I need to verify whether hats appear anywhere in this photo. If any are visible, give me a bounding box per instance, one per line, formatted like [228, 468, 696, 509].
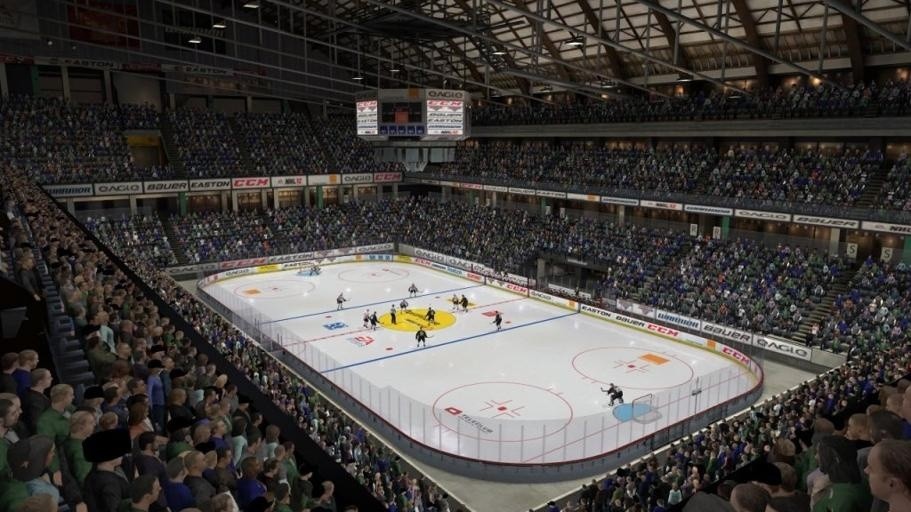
[6, 439, 43, 482]
[148, 360, 165, 368]
[169, 367, 188, 378]
[84, 324, 101, 336]
[151, 345, 166, 353]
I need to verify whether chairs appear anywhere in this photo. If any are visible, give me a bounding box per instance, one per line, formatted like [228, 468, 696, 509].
[1, 214, 96, 404]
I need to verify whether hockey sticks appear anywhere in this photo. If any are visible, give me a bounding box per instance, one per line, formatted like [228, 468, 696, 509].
[601, 387, 609, 392]
